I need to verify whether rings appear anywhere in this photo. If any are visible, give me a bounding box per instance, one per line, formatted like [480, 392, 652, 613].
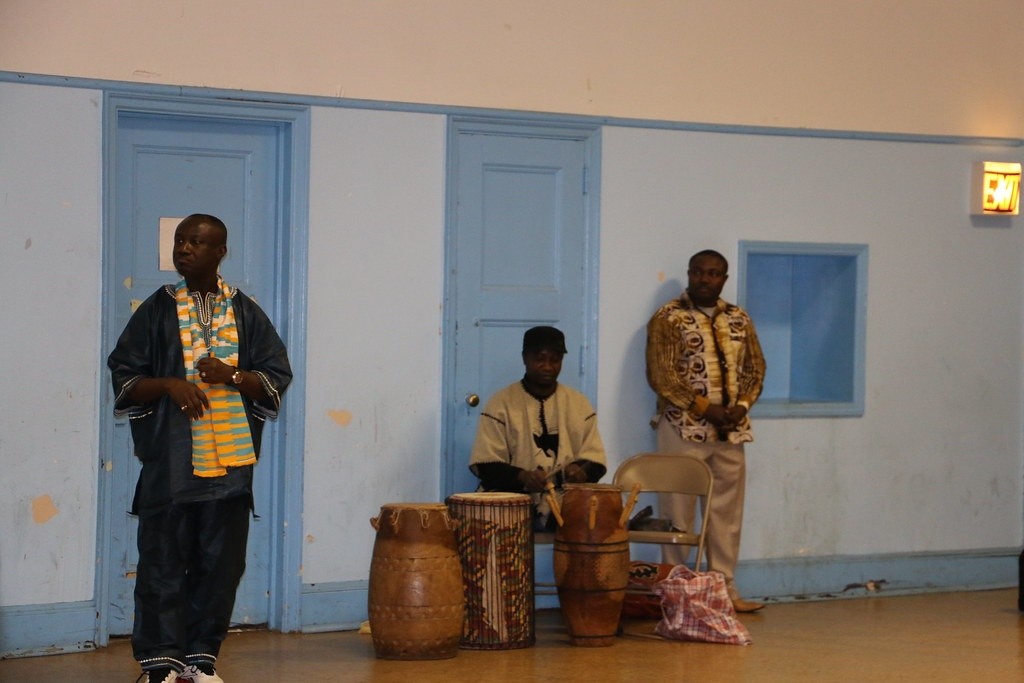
[201, 372, 206, 377]
[181, 404, 187, 410]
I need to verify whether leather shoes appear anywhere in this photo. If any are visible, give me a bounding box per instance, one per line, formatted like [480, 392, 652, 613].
[731, 598, 764, 613]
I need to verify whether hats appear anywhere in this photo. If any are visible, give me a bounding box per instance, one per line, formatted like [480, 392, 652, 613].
[522, 326, 570, 354]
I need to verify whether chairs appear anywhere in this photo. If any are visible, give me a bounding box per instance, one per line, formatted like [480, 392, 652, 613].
[610, 453, 713, 642]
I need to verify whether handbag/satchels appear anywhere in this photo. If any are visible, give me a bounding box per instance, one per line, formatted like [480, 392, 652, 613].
[653, 563, 753, 645]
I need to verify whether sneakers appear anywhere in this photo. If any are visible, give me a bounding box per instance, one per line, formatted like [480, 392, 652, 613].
[145, 664, 224, 683]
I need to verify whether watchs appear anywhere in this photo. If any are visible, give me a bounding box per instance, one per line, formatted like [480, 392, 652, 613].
[225, 365, 244, 387]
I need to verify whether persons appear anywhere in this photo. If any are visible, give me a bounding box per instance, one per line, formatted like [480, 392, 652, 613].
[469, 325, 608, 535]
[644, 249, 766, 612]
[106, 211, 294, 683]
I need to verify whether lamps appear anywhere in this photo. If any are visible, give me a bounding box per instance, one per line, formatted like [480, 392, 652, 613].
[969, 160, 1024, 217]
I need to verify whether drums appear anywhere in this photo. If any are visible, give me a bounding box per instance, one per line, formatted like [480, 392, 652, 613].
[364, 503, 465, 660]
[445, 491, 537, 650]
[541, 482, 642, 647]
